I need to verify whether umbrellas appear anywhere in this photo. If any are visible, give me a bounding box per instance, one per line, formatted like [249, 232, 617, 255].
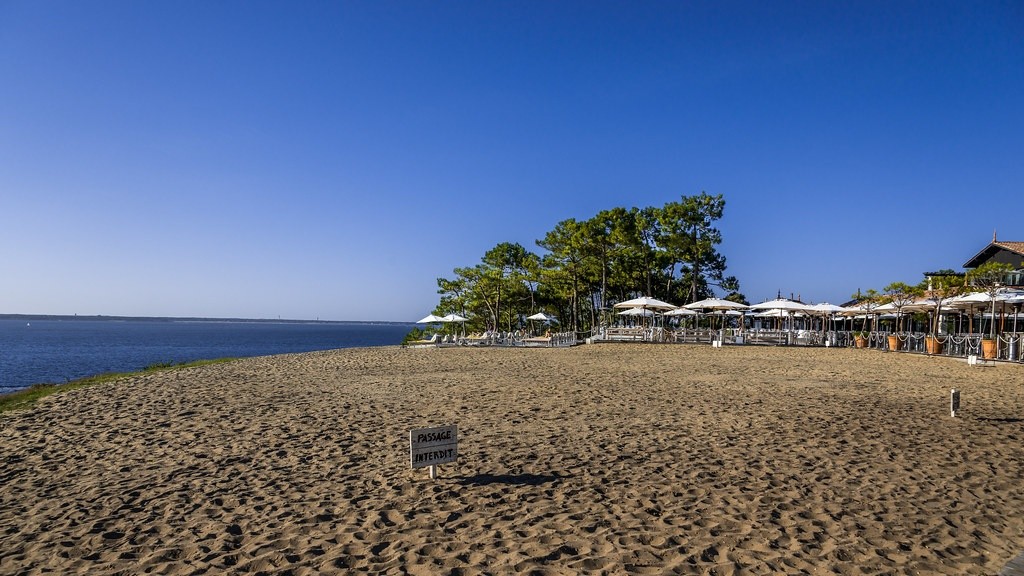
[614, 288, 1024, 338]
[526, 312, 553, 336]
[417, 313, 469, 334]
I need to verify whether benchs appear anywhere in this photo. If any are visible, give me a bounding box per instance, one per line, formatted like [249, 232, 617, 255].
[607, 328, 788, 345]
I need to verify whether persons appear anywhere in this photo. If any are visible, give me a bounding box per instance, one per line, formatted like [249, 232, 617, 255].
[545, 328, 551, 338]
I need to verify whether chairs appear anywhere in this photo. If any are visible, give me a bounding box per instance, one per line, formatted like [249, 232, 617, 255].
[407, 331, 577, 350]
